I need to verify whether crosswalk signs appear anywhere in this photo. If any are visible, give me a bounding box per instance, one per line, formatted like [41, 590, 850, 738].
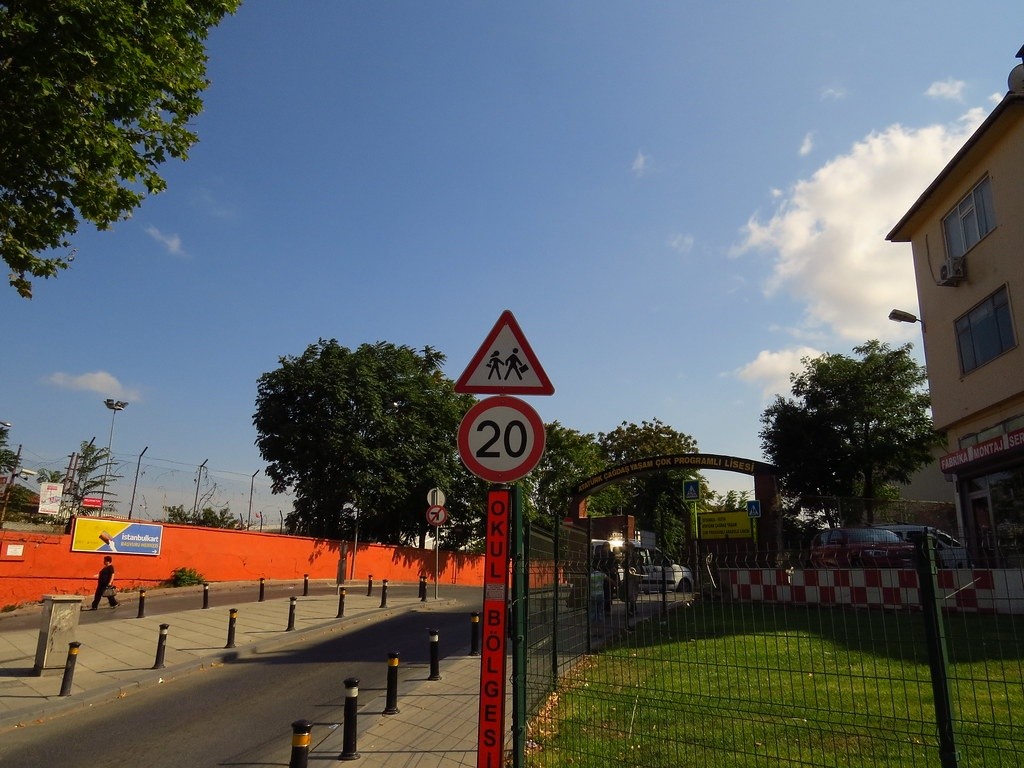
[747, 500, 760, 517]
[685, 480, 700, 501]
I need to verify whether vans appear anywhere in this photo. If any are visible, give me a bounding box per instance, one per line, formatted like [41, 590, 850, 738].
[873, 522, 985, 570]
[585, 539, 694, 593]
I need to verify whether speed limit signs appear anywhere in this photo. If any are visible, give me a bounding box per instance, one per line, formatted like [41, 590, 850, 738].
[455, 398, 547, 484]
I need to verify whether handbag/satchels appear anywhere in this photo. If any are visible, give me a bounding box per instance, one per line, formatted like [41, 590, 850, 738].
[102, 585, 116, 597]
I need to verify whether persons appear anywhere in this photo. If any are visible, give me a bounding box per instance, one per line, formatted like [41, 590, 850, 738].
[593, 542, 618, 617]
[90, 555, 121, 611]
[586, 561, 617, 638]
[622, 542, 644, 617]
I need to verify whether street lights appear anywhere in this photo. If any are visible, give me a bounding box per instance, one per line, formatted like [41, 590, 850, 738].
[129, 446, 147, 519]
[259, 512, 263, 532]
[246, 469, 260, 530]
[98, 399, 129, 517]
[193, 459, 208, 525]
[66, 437, 95, 531]
[239, 513, 243, 530]
[279, 510, 284, 535]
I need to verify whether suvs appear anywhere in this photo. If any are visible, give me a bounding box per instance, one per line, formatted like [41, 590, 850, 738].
[811, 525, 921, 569]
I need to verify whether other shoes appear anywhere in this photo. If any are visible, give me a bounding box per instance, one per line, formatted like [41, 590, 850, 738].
[628, 610, 633, 616]
[90, 607, 98, 610]
[112, 604, 119, 608]
